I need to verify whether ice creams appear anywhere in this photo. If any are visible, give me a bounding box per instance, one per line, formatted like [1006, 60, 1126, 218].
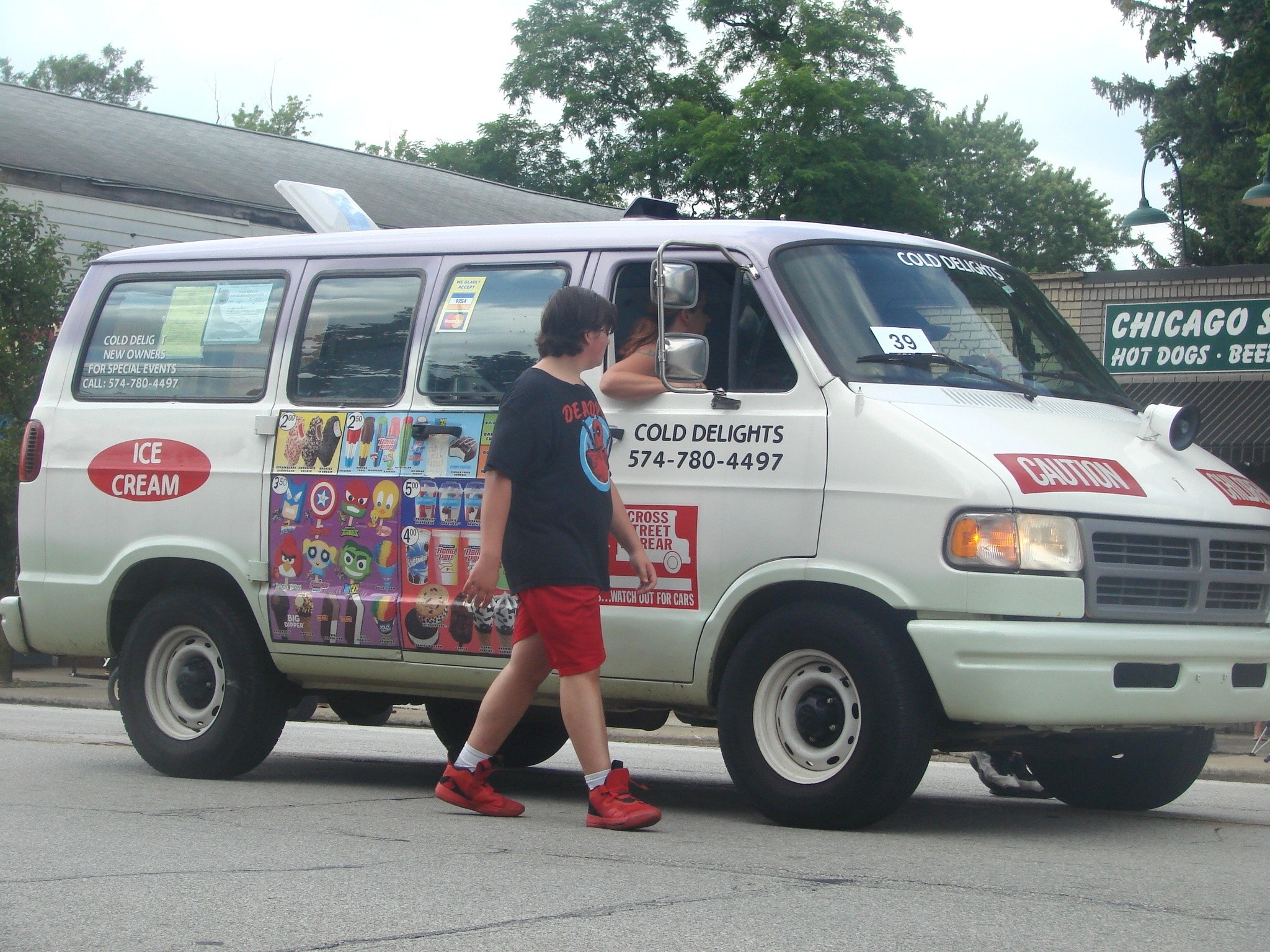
[469, 590, 518, 635]
[270, 590, 313, 642]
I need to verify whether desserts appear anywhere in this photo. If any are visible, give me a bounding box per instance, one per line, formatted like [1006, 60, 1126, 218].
[405, 608, 440, 647]
[449, 436, 478, 462]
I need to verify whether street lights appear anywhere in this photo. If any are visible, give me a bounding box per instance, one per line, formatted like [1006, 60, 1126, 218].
[1120, 145, 1188, 267]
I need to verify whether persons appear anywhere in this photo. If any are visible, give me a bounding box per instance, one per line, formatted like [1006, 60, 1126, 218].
[880, 306, 1003, 380]
[598, 260, 716, 401]
[434, 286, 664, 832]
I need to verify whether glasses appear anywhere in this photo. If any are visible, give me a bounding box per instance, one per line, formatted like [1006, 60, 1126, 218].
[695, 303, 711, 313]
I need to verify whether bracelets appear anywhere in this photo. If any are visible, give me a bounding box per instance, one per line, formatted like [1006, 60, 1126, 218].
[695, 384, 703, 389]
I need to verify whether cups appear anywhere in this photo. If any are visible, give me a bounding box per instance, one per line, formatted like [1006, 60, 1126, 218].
[438, 482, 465, 524]
[465, 481, 486, 527]
[496, 556, 511, 591]
[405, 528, 431, 585]
[459, 531, 483, 586]
[414, 479, 438, 523]
[431, 529, 460, 586]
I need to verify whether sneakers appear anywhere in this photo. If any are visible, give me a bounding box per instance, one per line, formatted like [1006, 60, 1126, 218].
[969, 750, 1051, 799]
[586, 760, 662, 832]
[434, 743, 525, 817]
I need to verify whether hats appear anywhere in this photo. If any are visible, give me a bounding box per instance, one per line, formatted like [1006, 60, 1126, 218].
[883, 307, 951, 343]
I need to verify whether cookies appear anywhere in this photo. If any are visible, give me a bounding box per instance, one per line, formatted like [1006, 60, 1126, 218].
[416, 584, 448, 617]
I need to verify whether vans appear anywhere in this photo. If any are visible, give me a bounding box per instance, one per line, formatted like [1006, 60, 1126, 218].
[1, 216, 1269, 830]
